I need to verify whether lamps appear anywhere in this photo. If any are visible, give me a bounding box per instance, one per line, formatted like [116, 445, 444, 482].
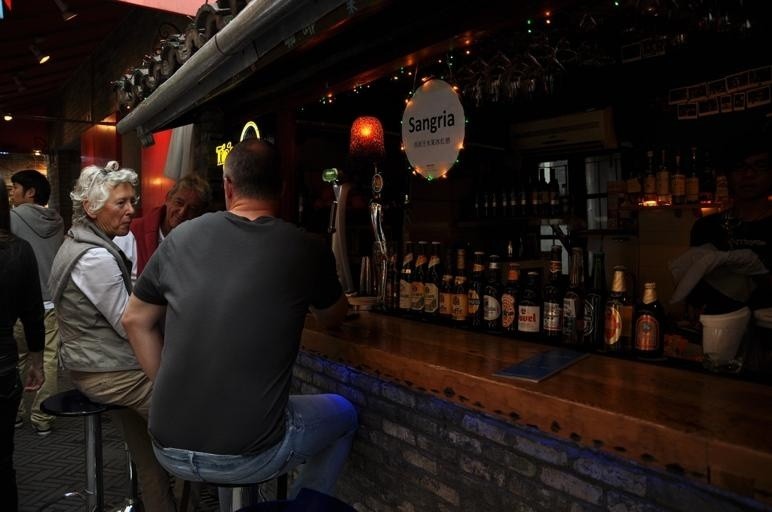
[1, 112, 15, 122]
[54, 0, 80, 22]
[27, 35, 50, 65]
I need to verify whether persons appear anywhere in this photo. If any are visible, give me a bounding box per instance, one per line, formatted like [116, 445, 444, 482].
[0, 177, 46, 512]
[112, 175, 213, 289]
[681, 130, 771, 371]
[48, 161, 202, 512]
[10, 170, 64, 435]
[121, 139, 359, 512]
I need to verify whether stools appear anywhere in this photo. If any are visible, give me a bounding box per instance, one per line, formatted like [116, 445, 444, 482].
[177, 472, 292, 512]
[35, 389, 145, 512]
[236, 487, 359, 512]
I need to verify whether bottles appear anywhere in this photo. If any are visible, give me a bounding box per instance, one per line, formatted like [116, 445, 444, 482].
[628, 145, 748, 208]
[393, 230, 663, 355]
[474, 166, 559, 210]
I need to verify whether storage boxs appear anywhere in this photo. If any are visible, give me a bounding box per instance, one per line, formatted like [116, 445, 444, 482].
[604, 208, 691, 322]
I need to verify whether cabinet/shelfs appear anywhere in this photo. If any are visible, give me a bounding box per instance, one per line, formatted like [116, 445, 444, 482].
[402, 107, 615, 269]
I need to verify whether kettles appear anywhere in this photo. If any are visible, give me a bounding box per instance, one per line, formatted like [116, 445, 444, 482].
[697, 304, 751, 362]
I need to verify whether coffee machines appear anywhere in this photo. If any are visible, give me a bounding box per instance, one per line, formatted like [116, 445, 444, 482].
[328, 164, 388, 311]
